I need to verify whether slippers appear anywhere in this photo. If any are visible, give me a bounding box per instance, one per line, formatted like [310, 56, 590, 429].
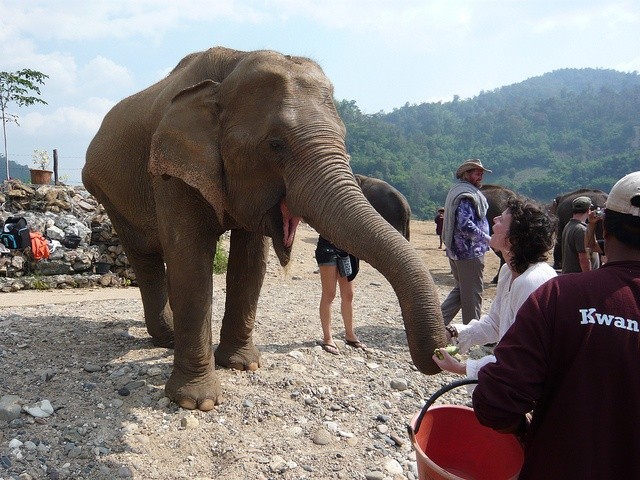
[321, 340, 339, 355]
[345, 339, 367, 349]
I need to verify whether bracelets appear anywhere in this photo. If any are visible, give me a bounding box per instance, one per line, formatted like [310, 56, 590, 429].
[446, 325, 459, 340]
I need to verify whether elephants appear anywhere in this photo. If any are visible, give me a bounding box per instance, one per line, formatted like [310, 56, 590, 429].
[554, 188, 611, 269]
[83, 47, 450, 413]
[354, 174, 411, 241]
[481, 184, 519, 284]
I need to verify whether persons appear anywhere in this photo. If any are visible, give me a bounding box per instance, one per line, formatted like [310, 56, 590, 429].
[471, 171, 640, 480]
[440, 158, 498, 325]
[434, 207, 447, 250]
[561, 195, 599, 273]
[314, 234, 368, 356]
[432, 197, 558, 397]
[588, 210, 607, 270]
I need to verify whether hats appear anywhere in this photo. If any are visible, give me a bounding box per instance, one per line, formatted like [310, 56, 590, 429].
[437, 207, 445, 212]
[604, 170, 640, 217]
[457, 159, 492, 176]
[571, 196, 594, 212]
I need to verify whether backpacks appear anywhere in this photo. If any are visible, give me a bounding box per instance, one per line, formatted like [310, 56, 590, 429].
[1, 215, 30, 249]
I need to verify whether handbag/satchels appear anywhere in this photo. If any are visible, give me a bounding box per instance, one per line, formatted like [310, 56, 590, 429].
[332, 249, 352, 278]
[60, 235, 82, 250]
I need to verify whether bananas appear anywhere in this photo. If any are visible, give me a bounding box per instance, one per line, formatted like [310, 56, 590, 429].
[434, 345, 461, 362]
